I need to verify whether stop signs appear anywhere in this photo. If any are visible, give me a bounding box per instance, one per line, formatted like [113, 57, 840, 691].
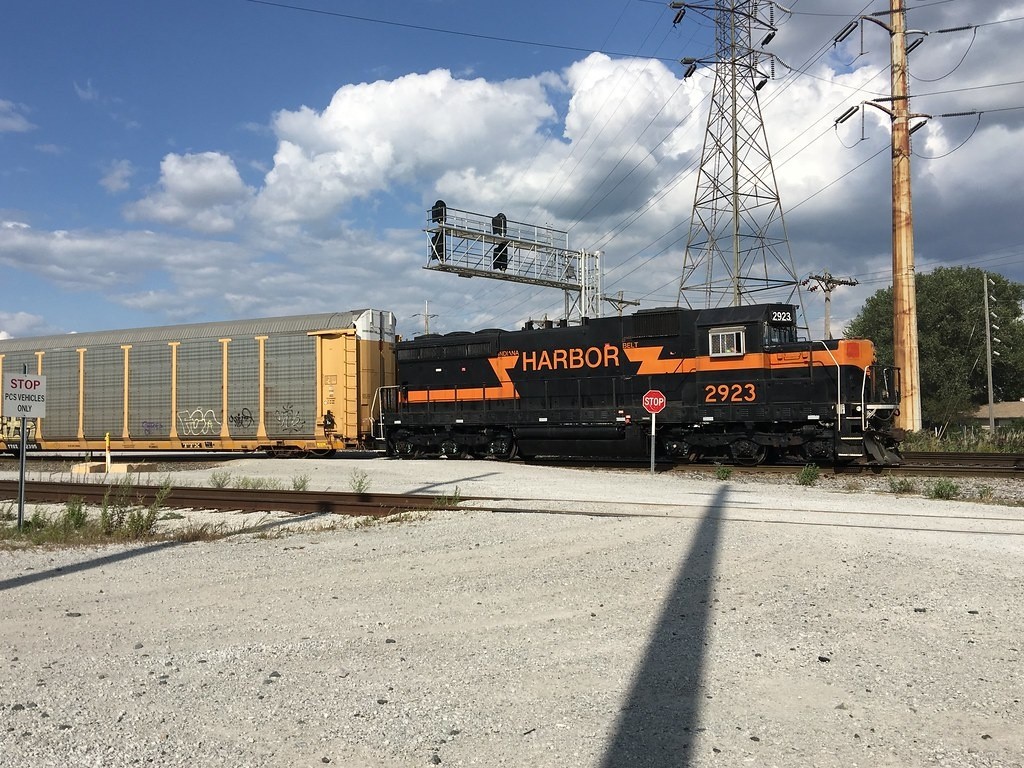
[643, 389, 666, 414]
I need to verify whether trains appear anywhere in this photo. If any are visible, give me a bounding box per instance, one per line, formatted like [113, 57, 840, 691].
[1, 302, 903, 473]
